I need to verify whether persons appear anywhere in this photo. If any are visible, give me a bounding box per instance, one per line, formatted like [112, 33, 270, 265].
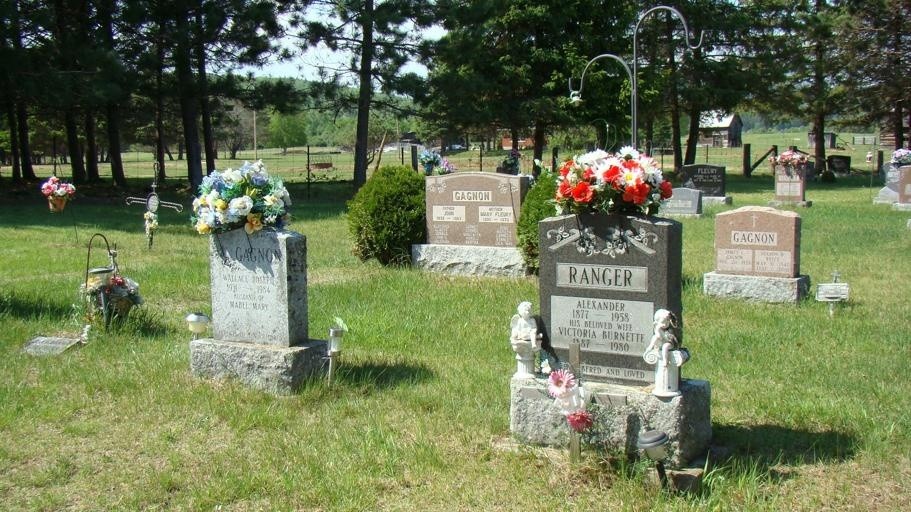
[507, 300, 544, 349]
[646, 307, 681, 368]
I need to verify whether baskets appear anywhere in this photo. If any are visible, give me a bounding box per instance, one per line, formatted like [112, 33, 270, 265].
[88, 293, 134, 321]
